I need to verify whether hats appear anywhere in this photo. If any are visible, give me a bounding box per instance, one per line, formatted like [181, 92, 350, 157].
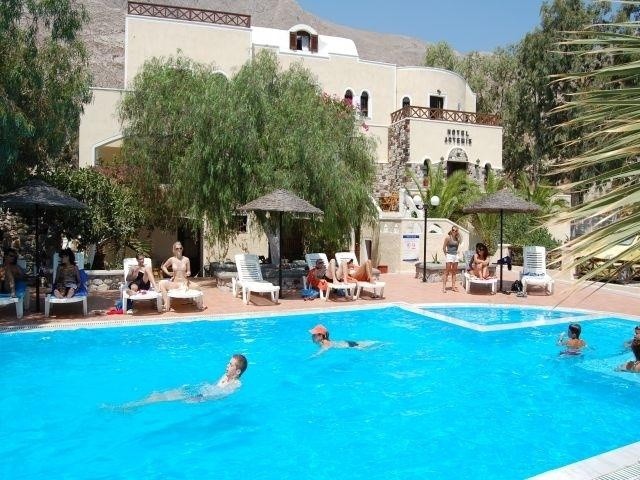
[309, 325, 327, 336]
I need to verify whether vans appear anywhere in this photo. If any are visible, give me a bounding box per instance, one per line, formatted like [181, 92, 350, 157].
[577, 234, 640, 283]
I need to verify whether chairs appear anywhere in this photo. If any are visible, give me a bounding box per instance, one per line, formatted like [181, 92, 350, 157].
[158, 280, 203, 311]
[119, 258, 162, 314]
[462, 251, 498, 293]
[302, 253, 356, 301]
[335, 252, 385, 300]
[519, 246, 554, 292]
[0, 260, 29, 319]
[45, 252, 87, 317]
[232, 254, 280, 305]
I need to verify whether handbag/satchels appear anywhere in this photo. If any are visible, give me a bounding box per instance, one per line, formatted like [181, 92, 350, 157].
[115, 298, 134, 311]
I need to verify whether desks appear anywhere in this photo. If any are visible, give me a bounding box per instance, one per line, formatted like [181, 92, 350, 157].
[415, 261, 467, 281]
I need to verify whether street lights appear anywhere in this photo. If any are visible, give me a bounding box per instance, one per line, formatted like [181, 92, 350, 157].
[413, 195, 440, 283]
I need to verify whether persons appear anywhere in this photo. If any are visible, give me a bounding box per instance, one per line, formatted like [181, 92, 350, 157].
[307, 325, 389, 359]
[558, 324, 586, 349]
[52, 248, 80, 299]
[346, 259, 376, 283]
[471, 243, 493, 279]
[0, 248, 25, 297]
[444, 229, 463, 291]
[126, 252, 158, 296]
[616, 326, 640, 375]
[101, 354, 246, 409]
[313, 259, 348, 284]
[157, 241, 191, 310]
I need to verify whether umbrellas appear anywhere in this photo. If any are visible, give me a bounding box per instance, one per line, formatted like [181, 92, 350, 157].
[236, 188, 325, 298]
[0, 179, 89, 314]
[463, 187, 543, 295]
